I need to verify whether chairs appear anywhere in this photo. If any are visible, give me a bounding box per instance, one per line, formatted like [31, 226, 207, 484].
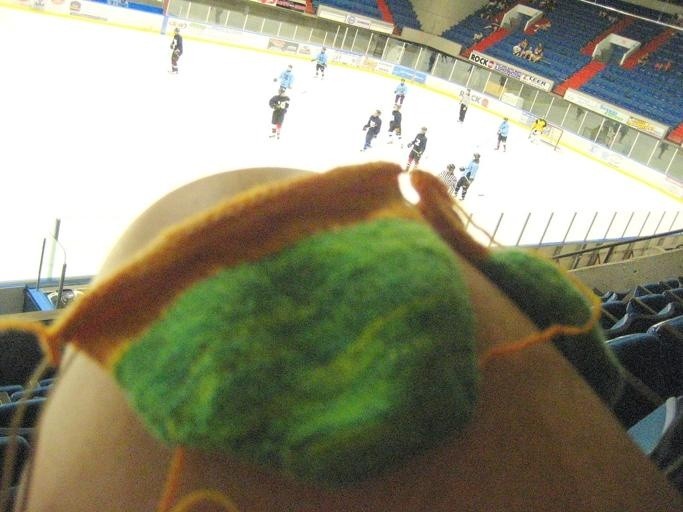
[591, 275, 683, 493]
[0, 377, 54, 508]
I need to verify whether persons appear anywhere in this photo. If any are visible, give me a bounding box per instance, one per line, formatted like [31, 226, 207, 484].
[528, 115, 547, 143]
[311, 48, 328, 79]
[437, 164, 457, 195]
[493, 117, 509, 153]
[454, 153, 481, 201]
[273, 64, 296, 89]
[359, 109, 382, 151]
[480, 0, 510, 33]
[512, 38, 544, 63]
[456, 89, 471, 123]
[406, 127, 428, 169]
[170, 26, 183, 73]
[269, 88, 290, 140]
[638, 52, 652, 66]
[394, 78, 407, 104]
[386, 104, 404, 150]
